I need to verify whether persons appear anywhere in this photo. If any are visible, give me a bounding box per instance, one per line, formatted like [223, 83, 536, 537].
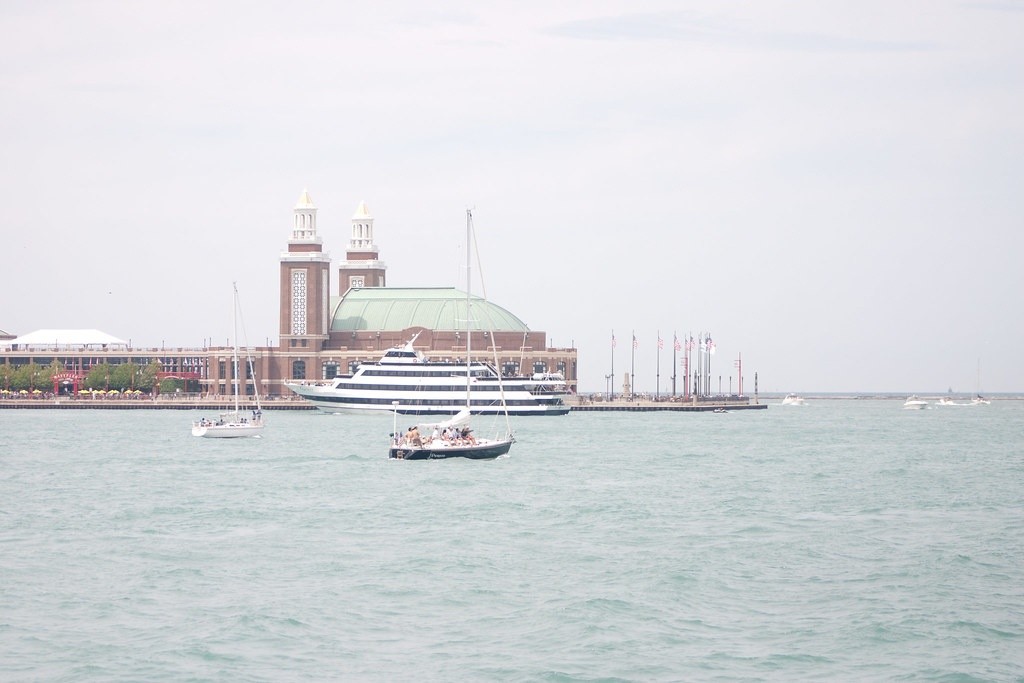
[239, 418, 244, 423]
[441, 424, 477, 446]
[256, 409, 263, 423]
[253, 411, 256, 420]
[432, 424, 440, 439]
[201, 418, 206, 427]
[405, 425, 428, 448]
[216, 419, 223, 426]
[244, 419, 248, 423]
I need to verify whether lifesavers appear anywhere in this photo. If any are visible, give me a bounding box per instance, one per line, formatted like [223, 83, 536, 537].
[413, 359, 417, 362]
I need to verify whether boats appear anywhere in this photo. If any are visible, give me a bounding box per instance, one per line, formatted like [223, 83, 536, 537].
[284, 332, 575, 416]
[904, 395, 928, 409]
[783, 394, 805, 405]
[940, 397, 954, 406]
[713, 409, 727, 413]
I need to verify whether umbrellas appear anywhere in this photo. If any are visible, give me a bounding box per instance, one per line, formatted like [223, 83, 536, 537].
[78, 389, 143, 400]
[1, 389, 42, 398]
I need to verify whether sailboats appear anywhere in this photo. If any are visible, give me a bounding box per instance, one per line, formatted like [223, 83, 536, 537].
[191, 283, 264, 438]
[970, 358, 990, 405]
[388, 208, 518, 462]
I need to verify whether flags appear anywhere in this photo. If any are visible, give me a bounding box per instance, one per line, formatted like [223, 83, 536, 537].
[674, 336, 681, 351]
[657, 337, 663, 349]
[612, 334, 616, 350]
[633, 335, 640, 350]
[684, 330, 717, 351]
